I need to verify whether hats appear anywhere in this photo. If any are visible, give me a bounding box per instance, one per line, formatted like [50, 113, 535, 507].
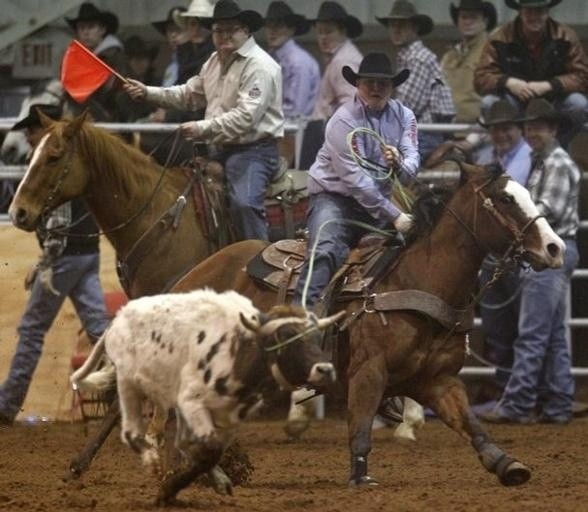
[64, 3, 120, 37]
[375, 0, 435, 35]
[12, 103, 63, 131]
[505, 0, 561, 10]
[450, 0, 498, 33]
[342, 52, 411, 90]
[476, 101, 519, 127]
[151, 1, 366, 40]
[513, 98, 561, 123]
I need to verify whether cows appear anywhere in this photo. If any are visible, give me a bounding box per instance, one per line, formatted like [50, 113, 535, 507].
[69, 287, 346, 507]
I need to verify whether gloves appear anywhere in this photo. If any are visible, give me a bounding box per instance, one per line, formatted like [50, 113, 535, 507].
[394, 212, 413, 234]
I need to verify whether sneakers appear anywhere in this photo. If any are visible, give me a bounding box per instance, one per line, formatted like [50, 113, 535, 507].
[477, 410, 570, 425]
[379, 396, 405, 422]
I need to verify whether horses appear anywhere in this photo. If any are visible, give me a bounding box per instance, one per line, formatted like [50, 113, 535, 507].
[8, 105, 425, 480]
[169, 156, 566, 488]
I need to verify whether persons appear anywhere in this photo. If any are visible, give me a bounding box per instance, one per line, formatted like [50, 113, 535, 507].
[65, 2, 130, 123]
[0, 103, 113, 428]
[123, 0, 283, 239]
[476, 102, 532, 402]
[291, 54, 420, 310]
[264, 0, 363, 170]
[473, 0, 588, 147]
[478, 100, 580, 424]
[127, 0, 213, 165]
[376, 0, 497, 160]
